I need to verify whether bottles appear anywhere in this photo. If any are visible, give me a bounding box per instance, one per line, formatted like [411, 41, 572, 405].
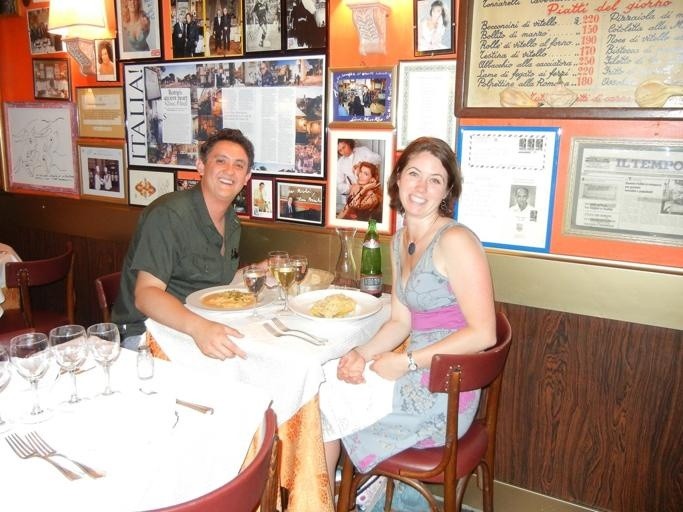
[359, 220, 382, 299]
[137, 345, 154, 380]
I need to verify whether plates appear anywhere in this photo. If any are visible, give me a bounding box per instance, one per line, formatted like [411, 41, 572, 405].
[186, 286, 276, 312]
[305, 269, 334, 283]
[288, 288, 382, 321]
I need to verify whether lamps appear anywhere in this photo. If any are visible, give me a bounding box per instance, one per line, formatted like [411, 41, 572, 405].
[47, 0, 110, 38]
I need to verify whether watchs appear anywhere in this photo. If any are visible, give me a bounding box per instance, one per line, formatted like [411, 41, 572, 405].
[406, 351, 418, 373]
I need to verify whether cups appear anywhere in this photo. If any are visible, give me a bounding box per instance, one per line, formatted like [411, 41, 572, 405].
[87, 323, 120, 395]
[243, 267, 266, 321]
[49, 325, 87, 404]
[0, 344, 11, 426]
[269, 250, 289, 305]
[291, 255, 308, 295]
[9, 334, 49, 415]
[278, 259, 297, 316]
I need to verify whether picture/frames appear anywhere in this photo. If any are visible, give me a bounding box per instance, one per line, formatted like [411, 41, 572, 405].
[115, 0, 164, 63]
[28, 8, 64, 53]
[249, 178, 276, 223]
[74, 143, 127, 203]
[74, 87, 125, 140]
[275, 178, 327, 228]
[93, 39, 120, 82]
[283, 0, 328, 53]
[244, 0, 281, 55]
[412, 0, 455, 56]
[128, 167, 175, 209]
[124, 54, 325, 179]
[32, 58, 71, 101]
[171, 0, 207, 61]
[0, 100, 79, 201]
[207, 1, 242, 58]
[329, 126, 397, 236]
[327, 66, 397, 131]
[454, 0, 683, 120]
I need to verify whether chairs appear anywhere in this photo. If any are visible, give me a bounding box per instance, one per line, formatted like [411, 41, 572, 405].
[338, 309, 512, 512]
[139, 408, 282, 512]
[99, 271, 125, 323]
[0, 243, 74, 340]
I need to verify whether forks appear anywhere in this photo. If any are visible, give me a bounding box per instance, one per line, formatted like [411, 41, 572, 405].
[24, 432, 102, 478]
[271, 317, 328, 343]
[263, 323, 320, 346]
[4, 433, 80, 480]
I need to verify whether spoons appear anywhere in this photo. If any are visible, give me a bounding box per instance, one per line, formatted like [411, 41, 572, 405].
[139, 387, 214, 415]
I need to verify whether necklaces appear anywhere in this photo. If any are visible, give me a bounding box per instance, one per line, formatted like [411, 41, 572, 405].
[405, 216, 439, 256]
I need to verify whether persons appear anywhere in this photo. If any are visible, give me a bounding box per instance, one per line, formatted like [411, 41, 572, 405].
[220, 7, 230, 52]
[184, 13, 199, 57]
[173, 13, 185, 58]
[102, 167, 111, 191]
[94, 166, 103, 190]
[112, 128, 253, 363]
[96, 39, 115, 76]
[253, 180, 273, 221]
[336, 139, 381, 196]
[252, 181, 267, 213]
[284, 195, 294, 218]
[336, 162, 383, 219]
[418, 1, 451, 51]
[120, 0, 149, 53]
[211, 9, 223, 53]
[318, 137, 498, 506]
[504, 186, 537, 232]
[250, 0, 268, 48]
